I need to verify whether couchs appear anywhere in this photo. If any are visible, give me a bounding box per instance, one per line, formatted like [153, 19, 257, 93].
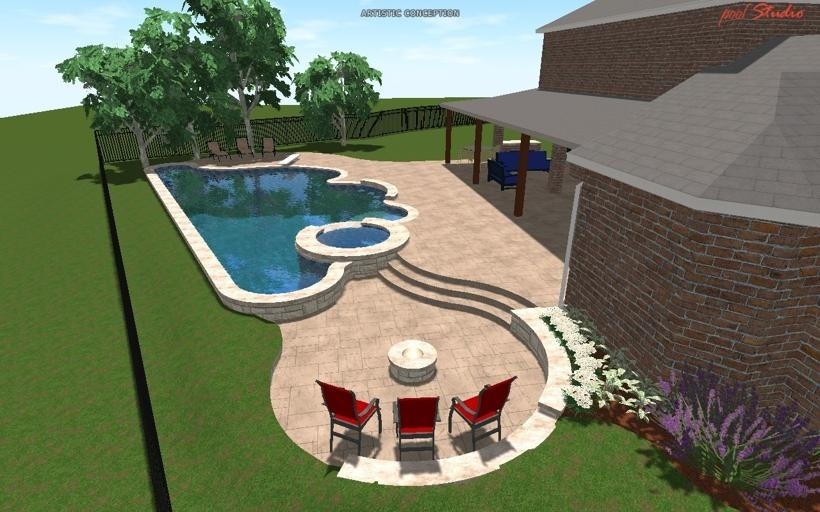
[486, 149, 552, 192]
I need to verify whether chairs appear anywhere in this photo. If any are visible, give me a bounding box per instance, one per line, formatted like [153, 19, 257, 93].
[206, 136, 276, 163]
[315, 374, 518, 462]
